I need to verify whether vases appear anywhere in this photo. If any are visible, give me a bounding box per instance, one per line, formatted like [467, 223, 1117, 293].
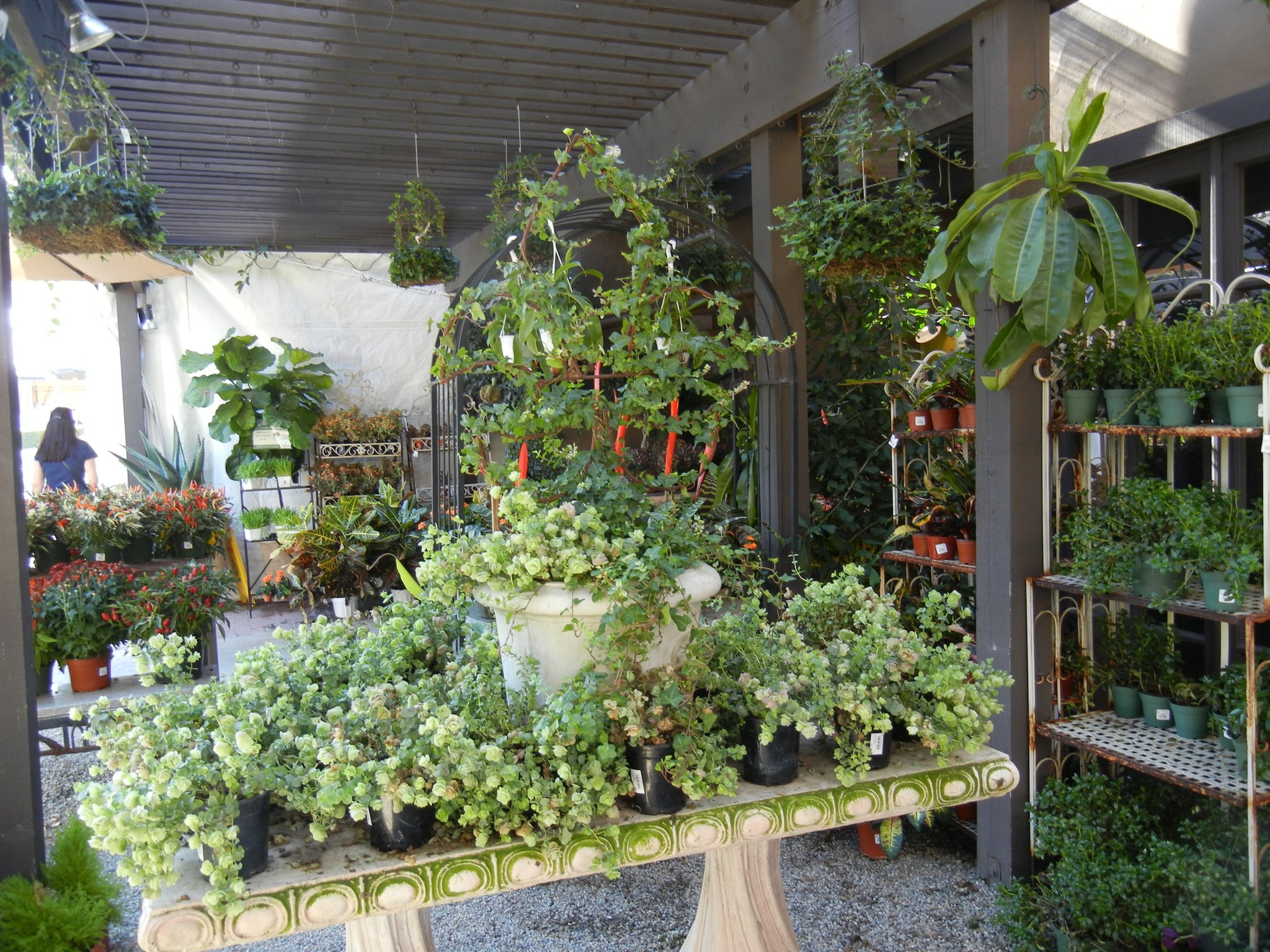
[356, 755, 437, 849]
[185, 784, 269, 878]
[479, 560, 722, 724]
[736, 717, 798, 786]
[281, 594, 286, 601]
[262, 592, 272, 602]
[67, 657, 111, 694]
[621, 735, 687, 815]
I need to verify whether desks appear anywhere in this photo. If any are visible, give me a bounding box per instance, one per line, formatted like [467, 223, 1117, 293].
[137, 732, 1019, 952]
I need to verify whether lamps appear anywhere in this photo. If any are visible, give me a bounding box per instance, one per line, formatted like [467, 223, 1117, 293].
[57, 0, 115, 52]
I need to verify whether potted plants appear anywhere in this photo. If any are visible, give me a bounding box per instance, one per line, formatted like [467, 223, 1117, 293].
[995, 302, 1270, 952]
[900, 350, 975, 565]
[768, 166, 948, 281]
[238, 405, 428, 619]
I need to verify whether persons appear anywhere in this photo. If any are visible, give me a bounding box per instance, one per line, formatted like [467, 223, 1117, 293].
[32, 406, 99, 495]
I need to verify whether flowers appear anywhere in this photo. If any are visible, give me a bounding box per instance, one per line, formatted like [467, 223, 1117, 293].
[31, 480, 1017, 885]
[275, 570, 292, 599]
[255, 574, 276, 596]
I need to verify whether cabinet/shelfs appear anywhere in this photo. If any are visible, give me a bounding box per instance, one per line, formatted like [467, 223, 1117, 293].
[881, 350, 975, 642]
[402, 424, 492, 536]
[239, 439, 320, 607]
[1016, 419, 1270, 952]
[314, 415, 403, 527]
